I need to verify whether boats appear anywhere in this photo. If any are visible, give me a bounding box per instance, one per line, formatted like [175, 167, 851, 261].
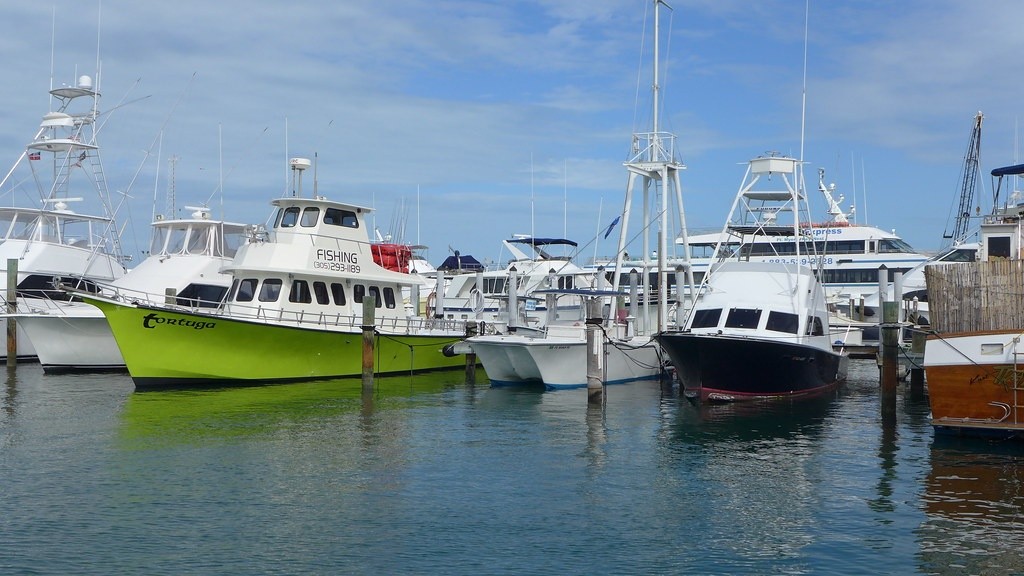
[56, 119, 485, 392]
[0, 0, 263, 376]
[428, 0, 983, 404]
